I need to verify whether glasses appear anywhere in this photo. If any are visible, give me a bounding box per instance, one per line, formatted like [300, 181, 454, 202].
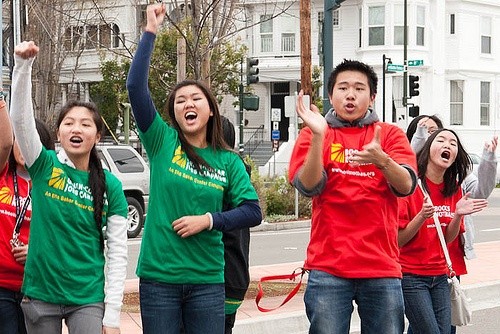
[430, 130, 437, 134]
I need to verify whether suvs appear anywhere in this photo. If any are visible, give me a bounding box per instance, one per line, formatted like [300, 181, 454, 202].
[96, 144, 153, 238]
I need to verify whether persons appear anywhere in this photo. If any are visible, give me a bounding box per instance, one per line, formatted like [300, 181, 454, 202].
[289, 60, 418, 334]
[406, 115, 498, 334]
[9, 41, 128, 334]
[127, 3, 263, 334]
[220, 115, 252, 334]
[0, 85, 51, 334]
[398, 129, 488, 334]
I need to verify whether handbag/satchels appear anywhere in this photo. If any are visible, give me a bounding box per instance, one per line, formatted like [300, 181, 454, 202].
[447, 276, 471, 326]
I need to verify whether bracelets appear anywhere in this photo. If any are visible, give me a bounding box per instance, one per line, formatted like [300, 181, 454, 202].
[376, 157, 391, 169]
[0, 91, 7, 108]
[207, 212, 213, 231]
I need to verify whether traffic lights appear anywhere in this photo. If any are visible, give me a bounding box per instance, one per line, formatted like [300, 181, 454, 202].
[408, 75, 420, 98]
[245, 56, 259, 86]
[118, 102, 132, 146]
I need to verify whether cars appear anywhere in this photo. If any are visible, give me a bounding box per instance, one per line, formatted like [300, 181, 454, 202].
[467, 151, 499, 187]
